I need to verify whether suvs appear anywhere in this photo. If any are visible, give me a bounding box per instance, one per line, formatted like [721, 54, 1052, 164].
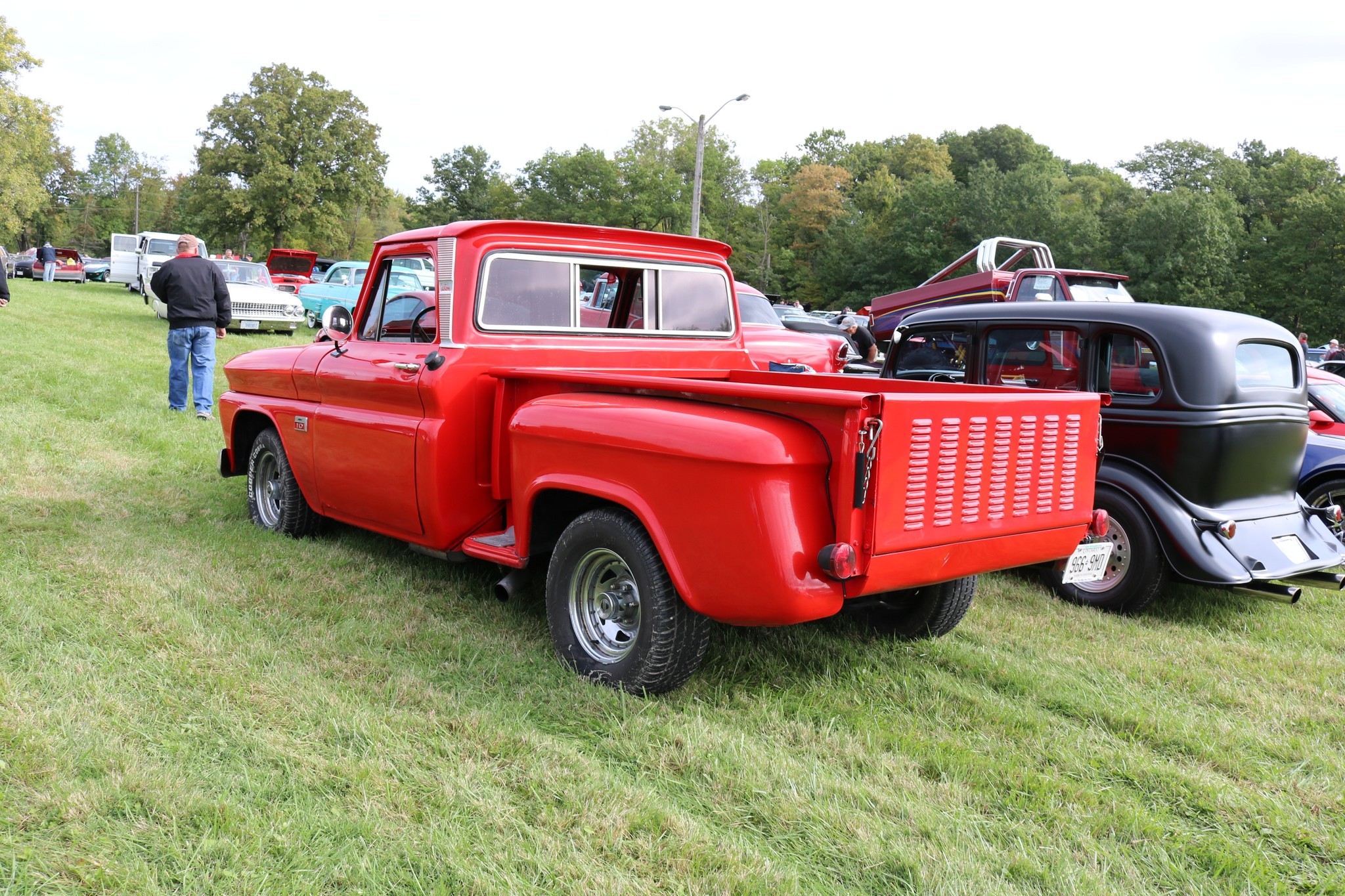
[867, 238, 1149, 391]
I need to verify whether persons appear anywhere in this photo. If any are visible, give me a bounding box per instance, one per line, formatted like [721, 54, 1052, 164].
[219, 249, 253, 283]
[1298, 333, 1345, 378]
[579, 274, 610, 300]
[0, 256, 10, 307]
[150, 233, 233, 419]
[39, 241, 57, 282]
[780, 299, 878, 363]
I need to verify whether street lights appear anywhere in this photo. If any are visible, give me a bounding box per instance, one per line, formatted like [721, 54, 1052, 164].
[659, 93, 750, 240]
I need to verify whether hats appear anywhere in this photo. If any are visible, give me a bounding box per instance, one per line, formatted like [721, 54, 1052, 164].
[1330, 339, 1338, 345]
[594, 274, 601, 280]
[178, 234, 202, 249]
[247, 252, 254, 258]
[44, 242, 50, 244]
[838, 316, 855, 330]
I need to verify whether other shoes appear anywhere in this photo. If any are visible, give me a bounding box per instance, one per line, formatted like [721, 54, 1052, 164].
[196, 412, 215, 419]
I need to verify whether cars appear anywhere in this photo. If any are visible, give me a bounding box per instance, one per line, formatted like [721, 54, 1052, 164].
[142, 258, 306, 338]
[209, 248, 437, 343]
[312, 271, 1345, 619]
[0, 247, 111, 284]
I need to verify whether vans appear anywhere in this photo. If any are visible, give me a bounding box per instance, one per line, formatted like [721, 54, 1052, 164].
[110, 231, 217, 299]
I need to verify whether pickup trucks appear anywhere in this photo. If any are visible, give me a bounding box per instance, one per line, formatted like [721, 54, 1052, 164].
[219, 221, 1104, 701]
